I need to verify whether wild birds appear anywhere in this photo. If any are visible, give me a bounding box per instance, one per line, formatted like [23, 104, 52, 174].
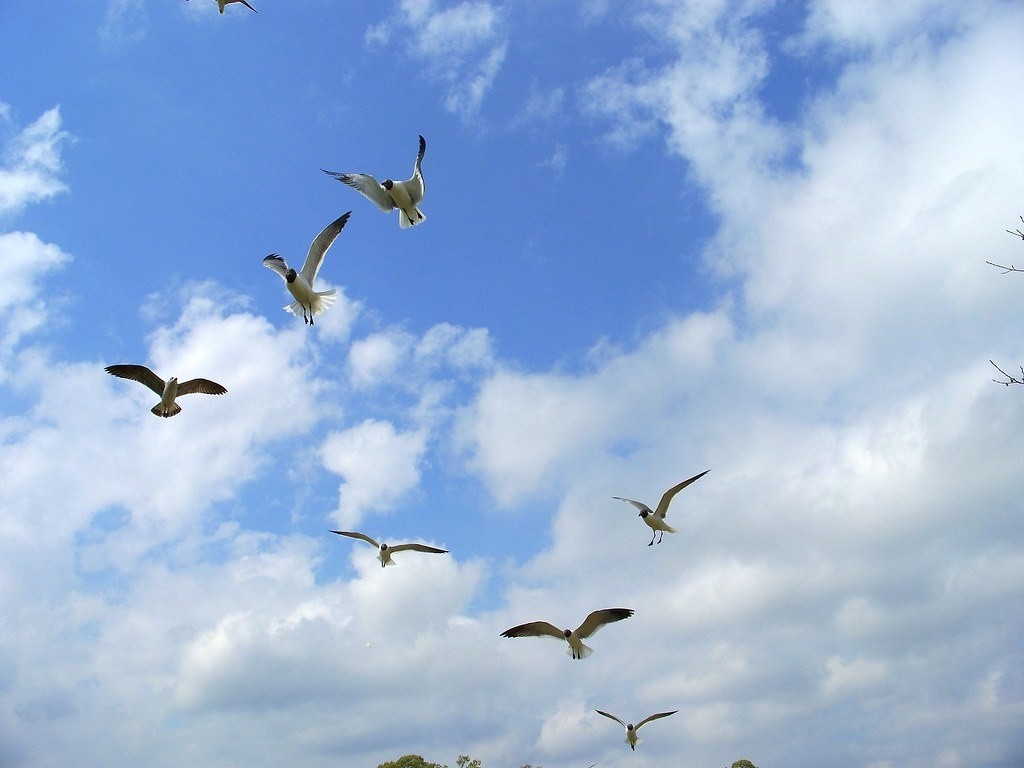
[328, 530, 450, 567]
[499, 470, 711, 751]
[104, 364, 228, 418]
[186, 0, 259, 14]
[263, 135, 427, 326]
